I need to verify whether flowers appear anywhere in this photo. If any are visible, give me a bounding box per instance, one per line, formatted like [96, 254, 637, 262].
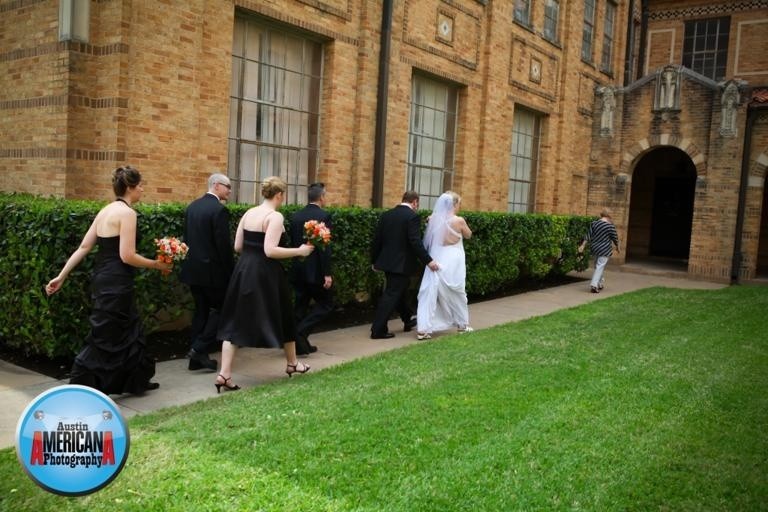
[151, 234, 190, 282]
[301, 216, 333, 256]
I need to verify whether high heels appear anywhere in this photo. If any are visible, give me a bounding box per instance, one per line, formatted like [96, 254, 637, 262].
[286, 362, 310, 377]
[215, 375, 240, 393]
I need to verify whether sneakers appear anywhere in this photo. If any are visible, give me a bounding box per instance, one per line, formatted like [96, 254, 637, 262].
[591, 286, 599, 292]
[299, 346, 317, 356]
[144, 381, 159, 390]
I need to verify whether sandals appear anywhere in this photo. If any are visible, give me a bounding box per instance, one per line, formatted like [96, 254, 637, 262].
[457, 325, 474, 334]
[417, 330, 431, 340]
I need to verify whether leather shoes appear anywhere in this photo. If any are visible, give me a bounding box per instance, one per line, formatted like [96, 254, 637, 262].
[371, 331, 394, 339]
[187, 347, 217, 369]
[405, 318, 417, 331]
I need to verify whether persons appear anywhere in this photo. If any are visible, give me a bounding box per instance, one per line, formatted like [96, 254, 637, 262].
[578, 209, 621, 292]
[214, 175, 316, 394]
[370, 190, 438, 339]
[44, 165, 174, 397]
[290, 182, 333, 355]
[417, 191, 473, 339]
[184, 173, 234, 370]
